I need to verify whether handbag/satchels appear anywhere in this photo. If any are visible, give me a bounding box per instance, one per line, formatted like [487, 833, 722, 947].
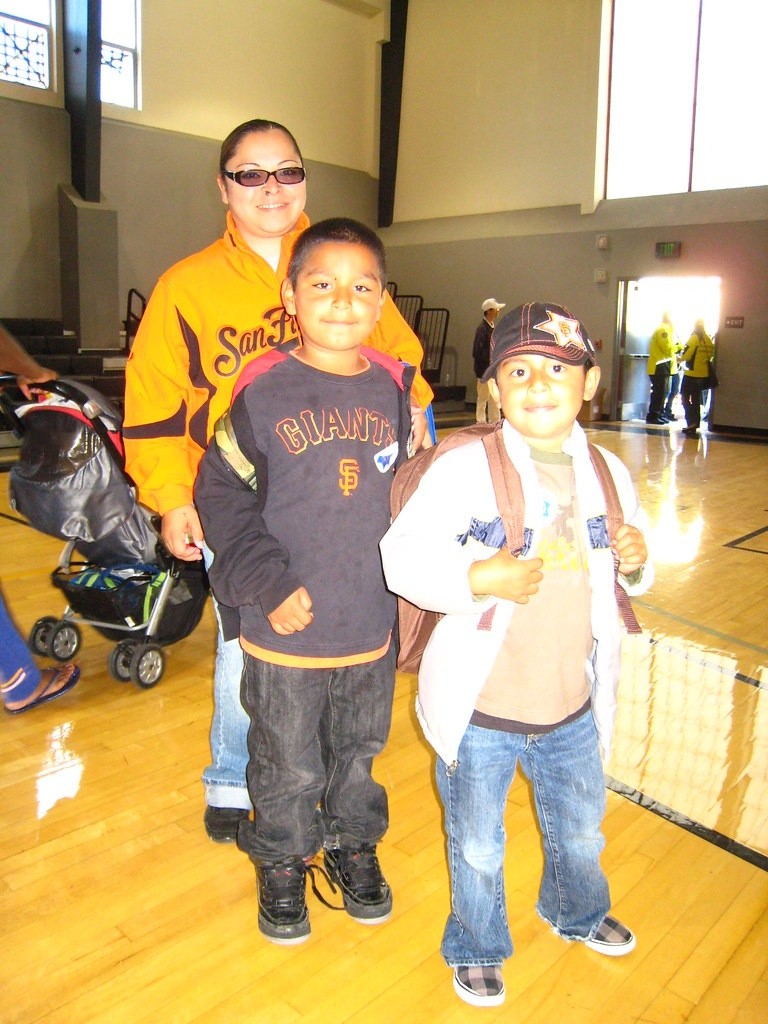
[703, 361, 719, 388]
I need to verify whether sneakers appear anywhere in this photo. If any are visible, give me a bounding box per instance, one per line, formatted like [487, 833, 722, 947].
[256, 852, 339, 946]
[323, 845, 392, 924]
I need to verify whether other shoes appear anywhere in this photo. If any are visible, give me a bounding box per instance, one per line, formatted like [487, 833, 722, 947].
[646, 413, 664, 424]
[455, 959, 508, 1008]
[660, 416, 669, 424]
[682, 424, 696, 434]
[583, 917, 637, 956]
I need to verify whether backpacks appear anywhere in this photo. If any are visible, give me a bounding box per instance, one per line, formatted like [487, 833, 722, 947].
[390, 424, 642, 674]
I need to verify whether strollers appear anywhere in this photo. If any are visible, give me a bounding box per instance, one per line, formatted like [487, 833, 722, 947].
[0, 375, 209, 689]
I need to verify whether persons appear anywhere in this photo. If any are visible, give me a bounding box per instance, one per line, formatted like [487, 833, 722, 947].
[378, 300, 657, 1007]
[647, 313, 716, 432]
[0, 326, 81, 714]
[192, 217, 425, 944]
[120, 119, 436, 845]
[473, 299, 506, 426]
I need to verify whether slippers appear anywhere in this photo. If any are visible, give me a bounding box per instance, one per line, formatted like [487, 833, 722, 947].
[5, 664, 80, 714]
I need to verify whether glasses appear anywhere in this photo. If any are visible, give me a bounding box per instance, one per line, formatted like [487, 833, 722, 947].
[221, 167, 307, 186]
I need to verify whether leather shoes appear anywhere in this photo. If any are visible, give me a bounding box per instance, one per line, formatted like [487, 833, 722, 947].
[205, 805, 250, 845]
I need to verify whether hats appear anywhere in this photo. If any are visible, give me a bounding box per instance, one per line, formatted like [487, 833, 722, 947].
[478, 300, 598, 385]
[481, 297, 506, 312]
